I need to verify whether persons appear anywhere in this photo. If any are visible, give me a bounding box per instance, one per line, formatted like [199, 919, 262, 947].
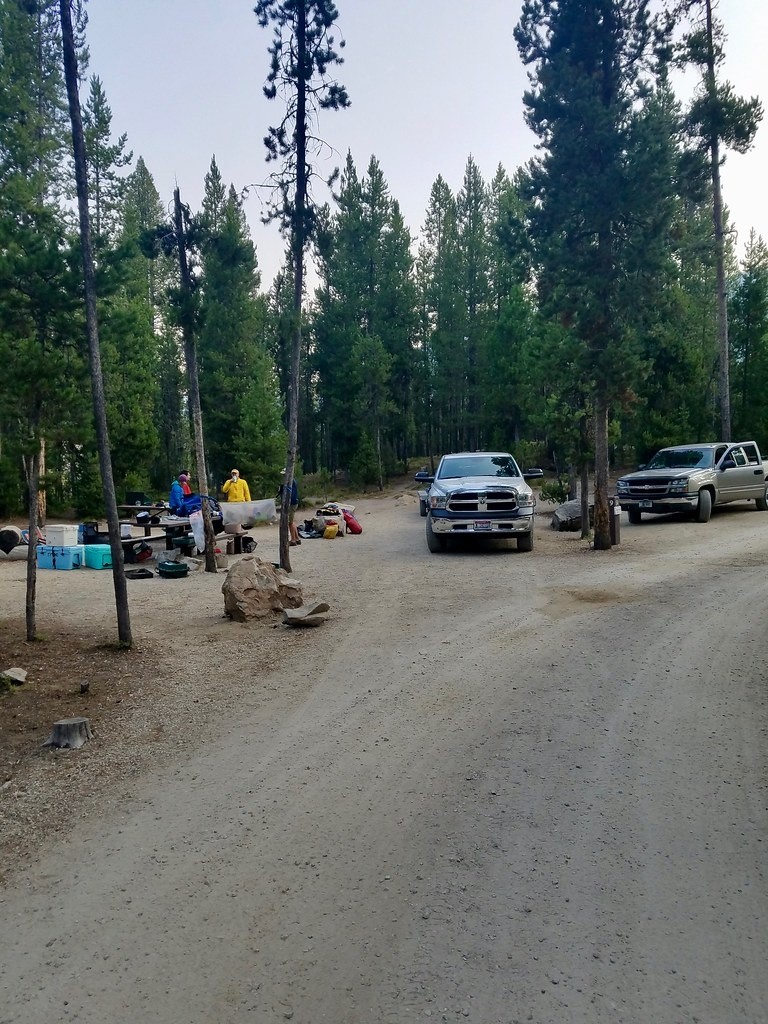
[223, 469, 251, 502]
[169, 469, 192, 507]
[278, 468, 301, 546]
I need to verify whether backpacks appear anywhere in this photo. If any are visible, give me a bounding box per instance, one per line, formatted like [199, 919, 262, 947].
[343, 509, 362, 534]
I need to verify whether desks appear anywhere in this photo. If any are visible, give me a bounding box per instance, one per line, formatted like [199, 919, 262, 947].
[116, 503, 170, 536]
[119, 516, 223, 550]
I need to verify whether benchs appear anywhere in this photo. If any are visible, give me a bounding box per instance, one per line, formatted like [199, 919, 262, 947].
[178, 531, 247, 557]
[121, 532, 180, 563]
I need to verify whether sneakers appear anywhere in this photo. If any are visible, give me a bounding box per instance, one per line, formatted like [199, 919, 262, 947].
[288, 540, 301, 546]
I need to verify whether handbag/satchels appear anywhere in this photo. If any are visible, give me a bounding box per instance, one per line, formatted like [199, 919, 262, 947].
[323, 524, 338, 539]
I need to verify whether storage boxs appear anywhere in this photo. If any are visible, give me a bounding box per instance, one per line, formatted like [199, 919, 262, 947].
[35, 524, 113, 569]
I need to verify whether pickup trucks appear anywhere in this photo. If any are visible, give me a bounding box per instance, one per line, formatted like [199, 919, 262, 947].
[614, 440, 768, 522]
[413, 451, 544, 553]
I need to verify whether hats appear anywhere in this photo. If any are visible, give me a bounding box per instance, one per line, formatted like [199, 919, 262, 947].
[179, 474, 187, 482]
[279, 467, 287, 474]
[231, 469, 239, 474]
